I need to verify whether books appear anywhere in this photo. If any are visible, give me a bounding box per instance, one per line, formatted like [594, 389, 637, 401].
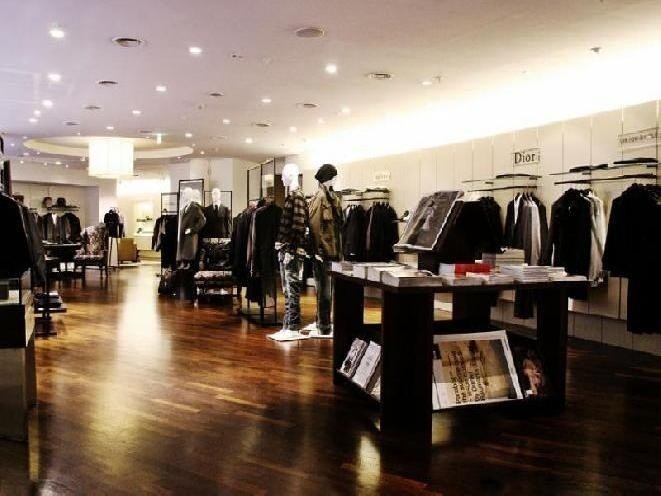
[338, 337, 382, 401]
[331, 247, 588, 290]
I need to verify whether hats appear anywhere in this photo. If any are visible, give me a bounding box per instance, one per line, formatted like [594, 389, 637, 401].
[316, 164, 337, 183]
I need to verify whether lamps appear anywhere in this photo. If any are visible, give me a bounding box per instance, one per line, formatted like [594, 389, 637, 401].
[88, 138, 134, 179]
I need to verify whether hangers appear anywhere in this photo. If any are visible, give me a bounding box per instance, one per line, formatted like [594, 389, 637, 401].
[515, 185, 535, 199]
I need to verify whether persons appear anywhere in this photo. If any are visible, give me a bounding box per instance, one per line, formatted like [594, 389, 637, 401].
[192, 190, 203, 209]
[203, 188, 231, 238]
[266, 164, 310, 343]
[176, 187, 207, 270]
[308, 162, 347, 337]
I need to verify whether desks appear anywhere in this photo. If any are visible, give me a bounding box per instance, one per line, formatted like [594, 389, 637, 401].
[327, 270, 591, 445]
